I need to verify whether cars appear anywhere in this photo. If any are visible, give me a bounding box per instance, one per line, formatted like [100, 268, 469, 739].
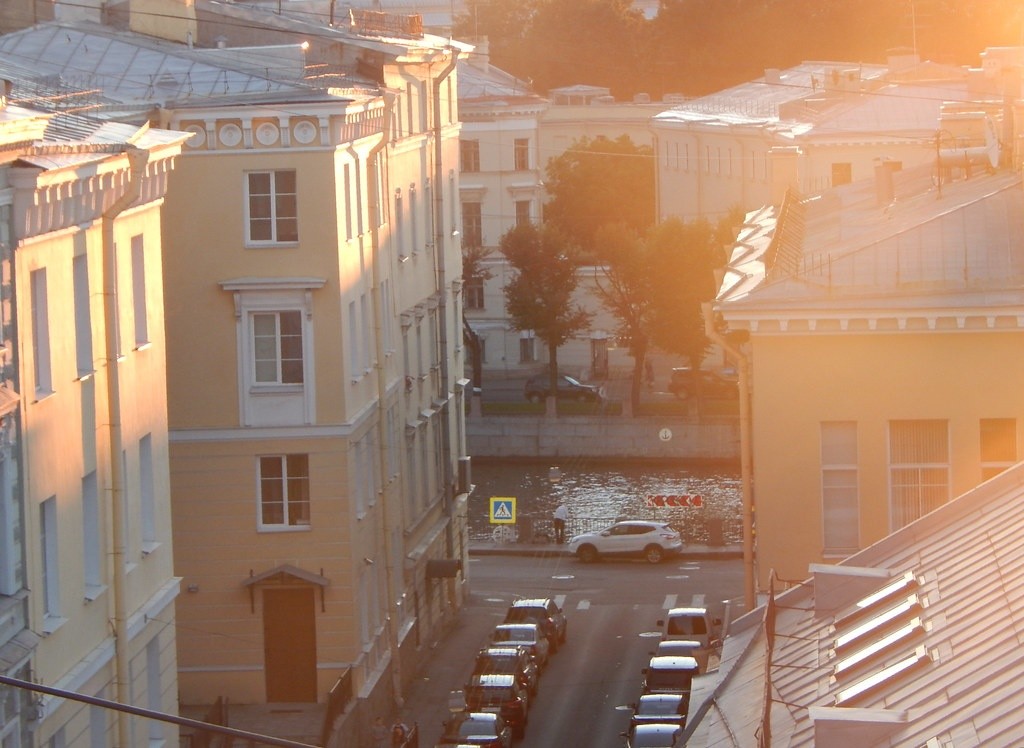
[617, 608, 722, 748]
[489, 623, 550, 667]
[433, 713, 514, 748]
[466, 674, 527, 739]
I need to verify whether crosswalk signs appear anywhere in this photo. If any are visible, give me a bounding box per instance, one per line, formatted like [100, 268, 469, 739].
[493, 501, 512, 520]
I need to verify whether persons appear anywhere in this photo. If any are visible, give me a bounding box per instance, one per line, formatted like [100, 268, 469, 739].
[644, 361, 656, 396]
[598, 382, 608, 416]
[390, 717, 410, 748]
[553, 503, 568, 546]
[371, 717, 388, 748]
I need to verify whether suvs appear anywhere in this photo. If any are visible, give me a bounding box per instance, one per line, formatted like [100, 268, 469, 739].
[567, 520, 684, 564]
[503, 597, 567, 653]
[667, 367, 738, 402]
[473, 645, 538, 708]
[524, 373, 609, 402]
